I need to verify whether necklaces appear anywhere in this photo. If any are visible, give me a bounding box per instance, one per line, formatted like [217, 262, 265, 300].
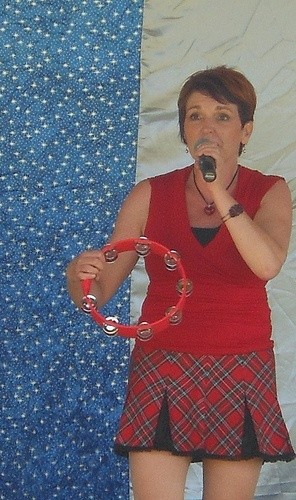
[192, 163, 241, 216]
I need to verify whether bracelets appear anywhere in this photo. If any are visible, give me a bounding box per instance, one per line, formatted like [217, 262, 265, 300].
[221, 204, 245, 224]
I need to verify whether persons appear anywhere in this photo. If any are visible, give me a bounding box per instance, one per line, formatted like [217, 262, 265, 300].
[66, 63, 296, 499]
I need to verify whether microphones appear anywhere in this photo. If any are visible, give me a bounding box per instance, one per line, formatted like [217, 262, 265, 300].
[195, 138, 217, 183]
